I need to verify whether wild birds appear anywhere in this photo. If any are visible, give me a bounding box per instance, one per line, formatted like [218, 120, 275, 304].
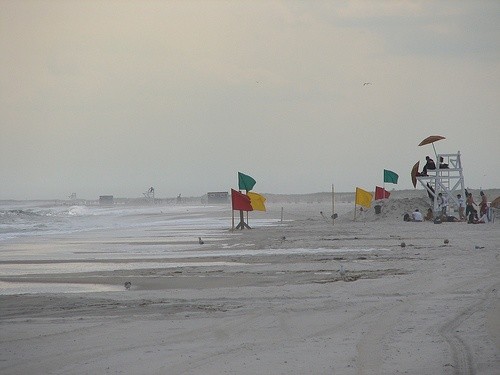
[198, 236, 203, 244]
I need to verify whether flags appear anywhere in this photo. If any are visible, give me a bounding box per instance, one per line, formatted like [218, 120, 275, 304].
[383, 169, 400, 184]
[375, 186, 391, 200]
[356, 188, 373, 208]
[238, 172, 256, 191]
[232, 189, 253, 211]
[248, 192, 267, 211]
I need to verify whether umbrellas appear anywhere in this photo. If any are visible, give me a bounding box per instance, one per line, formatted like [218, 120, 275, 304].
[417, 135, 446, 158]
[411, 160, 420, 188]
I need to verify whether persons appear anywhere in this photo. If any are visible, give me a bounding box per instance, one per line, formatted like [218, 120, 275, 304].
[421, 155, 436, 175]
[438, 193, 446, 215]
[412, 209, 424, 222]
[424, 208, 432, 222]
[467, 210, 488, 224]
[479, 191, 487, 218]
[457, 194, 465, 219]
[465, 189, 469, 198]
[466, 192, 479, 220]
